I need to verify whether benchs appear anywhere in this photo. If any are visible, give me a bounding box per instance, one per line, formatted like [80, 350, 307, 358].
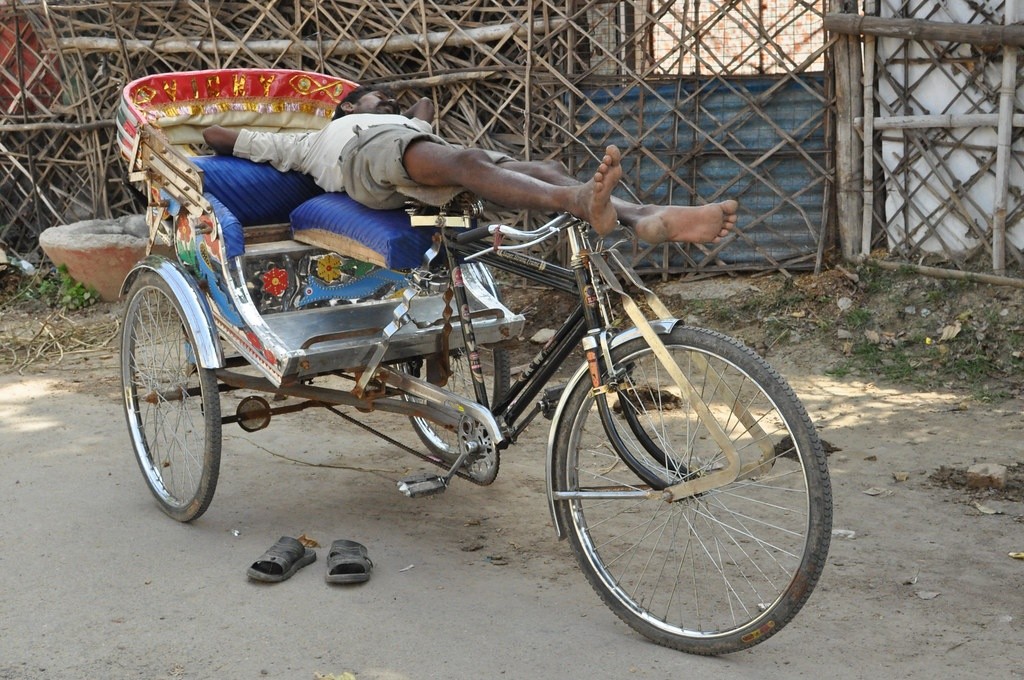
[181, 139, 335, 260]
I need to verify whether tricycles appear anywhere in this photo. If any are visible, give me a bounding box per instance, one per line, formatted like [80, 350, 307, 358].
[115, 67, 835, 655]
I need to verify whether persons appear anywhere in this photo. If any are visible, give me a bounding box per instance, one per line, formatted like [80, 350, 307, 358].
[203, 82, 740, 244]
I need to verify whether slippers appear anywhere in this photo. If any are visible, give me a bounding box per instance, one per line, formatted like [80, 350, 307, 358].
[246, 535, 316, 582]
[325, 539, 373, 583]
